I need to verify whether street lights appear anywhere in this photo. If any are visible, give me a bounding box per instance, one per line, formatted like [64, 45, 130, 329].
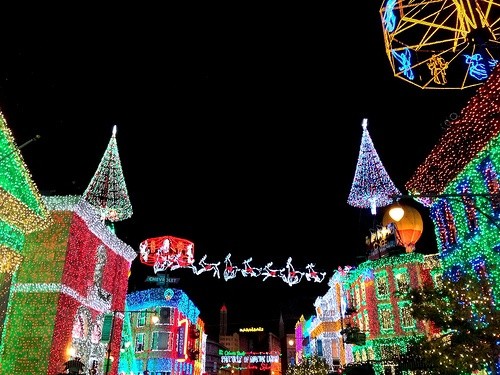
[288, 337, 318, 363]
[106, 309, 159, 375]
[387, 192, 499, 230]
